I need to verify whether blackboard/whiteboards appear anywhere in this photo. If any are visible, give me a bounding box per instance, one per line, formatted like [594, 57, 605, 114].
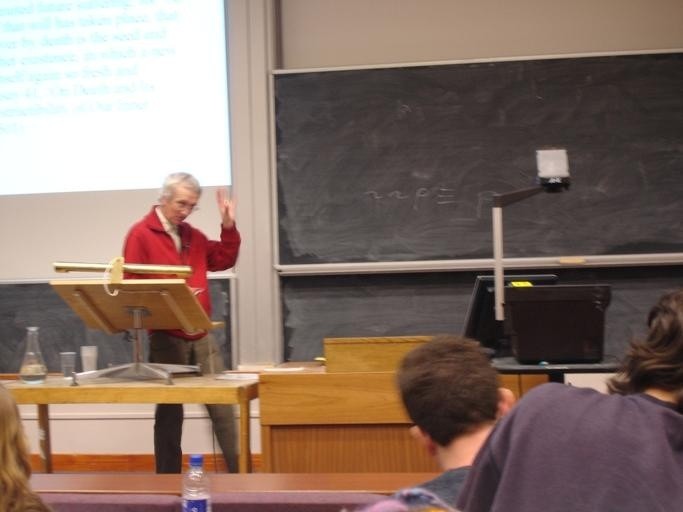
[267, 50, 682, 276]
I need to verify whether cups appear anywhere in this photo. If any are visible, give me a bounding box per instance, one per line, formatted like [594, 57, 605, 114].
[60, 345, 97, 378]
[205, 320, 227, 380]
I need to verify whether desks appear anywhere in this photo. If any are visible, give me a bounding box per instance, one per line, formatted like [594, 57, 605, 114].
[0, 377, 258, 474]
[24, 470, 449, 510]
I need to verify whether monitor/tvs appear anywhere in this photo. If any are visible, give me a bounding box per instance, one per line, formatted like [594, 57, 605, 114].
[460, 275, 558, 358]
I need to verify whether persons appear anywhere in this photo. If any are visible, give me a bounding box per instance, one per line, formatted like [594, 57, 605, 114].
[0, 384, 50, 512]
[124, 172, 242, 474]
[453, 287, 683, 511]
[358, 334, 514, 512]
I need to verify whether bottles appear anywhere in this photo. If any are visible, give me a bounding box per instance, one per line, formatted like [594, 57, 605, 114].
[181, 454, 212, 512]
[19, 325, 47, 386]
